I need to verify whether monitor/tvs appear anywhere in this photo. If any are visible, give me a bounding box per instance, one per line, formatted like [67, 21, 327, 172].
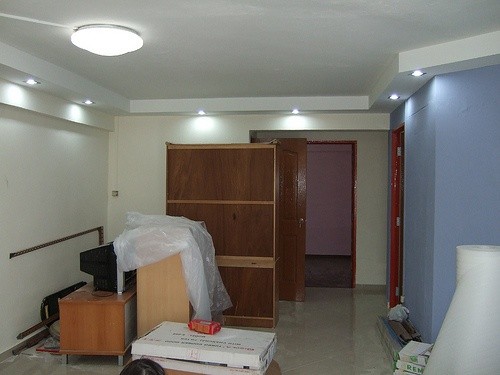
[80, 241, 137, 292]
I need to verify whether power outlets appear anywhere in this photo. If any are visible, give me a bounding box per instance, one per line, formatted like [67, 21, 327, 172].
[111, 190, 119, 200]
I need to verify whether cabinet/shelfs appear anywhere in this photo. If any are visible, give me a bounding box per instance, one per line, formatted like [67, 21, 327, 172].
[58, 138, 281, 367]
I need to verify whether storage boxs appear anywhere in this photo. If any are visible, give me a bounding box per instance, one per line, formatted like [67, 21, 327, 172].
[131, 321, 278, 375]
[188, 319, 221, 335]
[375, 316, 434, 375]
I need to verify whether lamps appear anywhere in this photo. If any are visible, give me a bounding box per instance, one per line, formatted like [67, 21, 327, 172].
[71, 25, 143, 57]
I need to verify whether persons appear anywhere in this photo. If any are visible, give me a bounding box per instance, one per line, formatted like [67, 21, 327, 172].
[119, 357, 166, 375]
